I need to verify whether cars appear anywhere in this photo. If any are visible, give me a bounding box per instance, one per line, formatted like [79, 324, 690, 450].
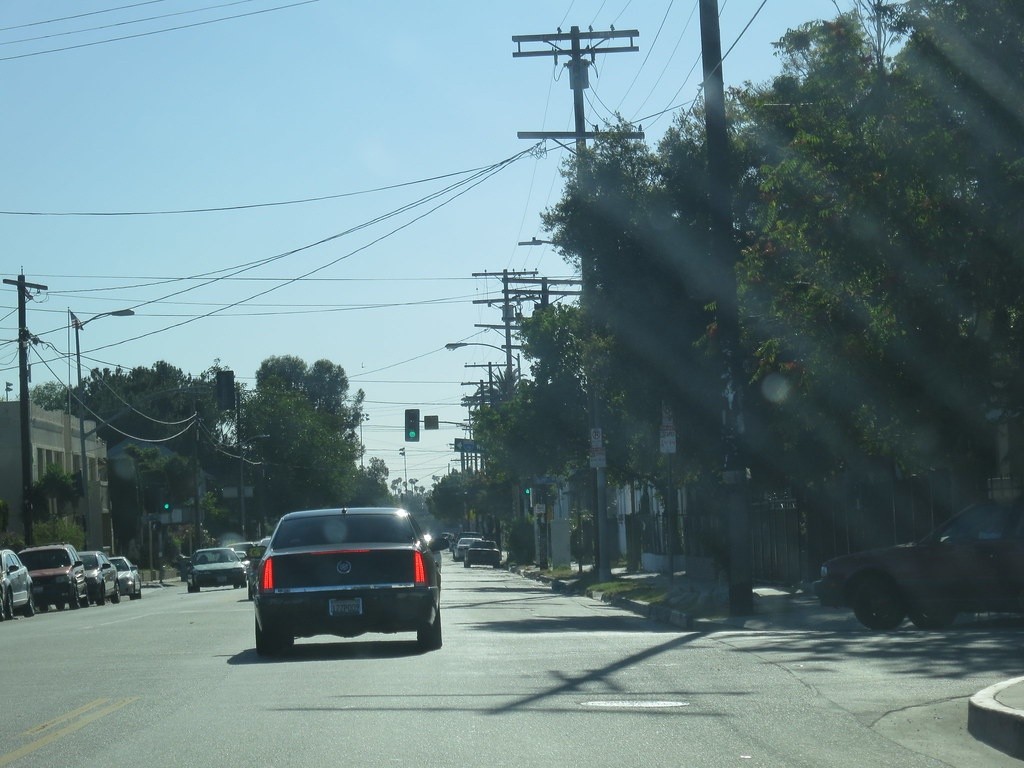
[246, 504, 449, 657]
[447, 532, 501, 569]
[186, 536, 272, 600]
[77, 550, 122, 606]
[109, 556, 142, 601]
[0, 549, 37, 621]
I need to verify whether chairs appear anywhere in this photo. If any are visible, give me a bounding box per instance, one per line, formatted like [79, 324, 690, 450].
[199, 555, 208, 563]
[218, 552, 230, 562]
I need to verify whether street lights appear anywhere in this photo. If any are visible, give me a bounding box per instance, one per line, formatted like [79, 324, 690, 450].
[67, 308, 136, 548]
[444, 341, 522, 404]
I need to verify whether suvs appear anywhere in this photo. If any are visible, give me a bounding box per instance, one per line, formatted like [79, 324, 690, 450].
[17, 542, 91, 613]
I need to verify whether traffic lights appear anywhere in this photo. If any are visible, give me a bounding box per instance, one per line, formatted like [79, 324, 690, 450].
[423, 415, 438, 429]
[404, 409, 420, 443]
